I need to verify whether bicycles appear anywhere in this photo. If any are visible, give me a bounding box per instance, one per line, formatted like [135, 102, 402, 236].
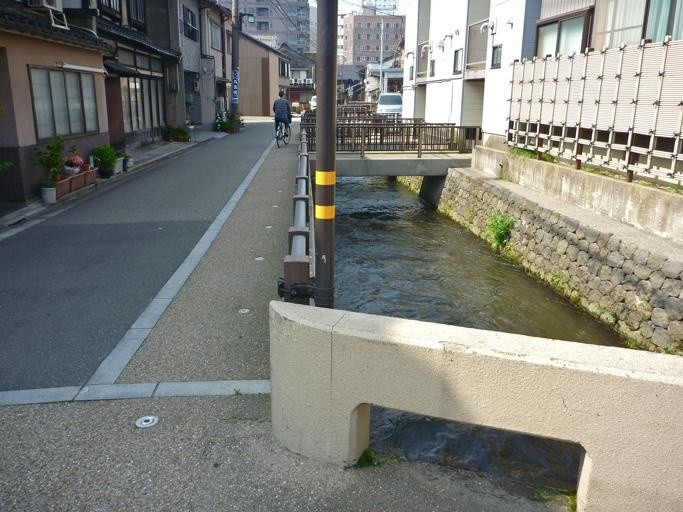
[215, 112, 223, 131]
[274, 114, 292, 148]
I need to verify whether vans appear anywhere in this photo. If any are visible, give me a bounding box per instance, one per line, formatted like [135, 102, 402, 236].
[376, 92, 403, 122]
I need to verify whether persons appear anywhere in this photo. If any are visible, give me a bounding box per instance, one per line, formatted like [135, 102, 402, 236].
[272, 90, 291, 137]
[309, 94, 316, 112]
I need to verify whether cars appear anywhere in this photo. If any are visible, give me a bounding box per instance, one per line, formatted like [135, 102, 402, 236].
[309, 95, 316, 111]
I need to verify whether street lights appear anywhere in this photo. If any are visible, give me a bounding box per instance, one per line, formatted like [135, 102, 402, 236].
[231, 10, 255, 128]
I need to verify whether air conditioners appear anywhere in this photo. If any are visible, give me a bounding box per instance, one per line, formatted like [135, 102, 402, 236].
[290, 79, 312, 84]
[27, 0, 63, 13]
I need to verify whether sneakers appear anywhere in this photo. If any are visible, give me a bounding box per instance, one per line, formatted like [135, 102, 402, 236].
[284, 130, 289, 137]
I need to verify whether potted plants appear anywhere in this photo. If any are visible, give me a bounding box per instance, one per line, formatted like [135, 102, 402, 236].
[33, 135, 125, 204]
[168, 127, 190, 142]
[216, 112, 243, 134]
